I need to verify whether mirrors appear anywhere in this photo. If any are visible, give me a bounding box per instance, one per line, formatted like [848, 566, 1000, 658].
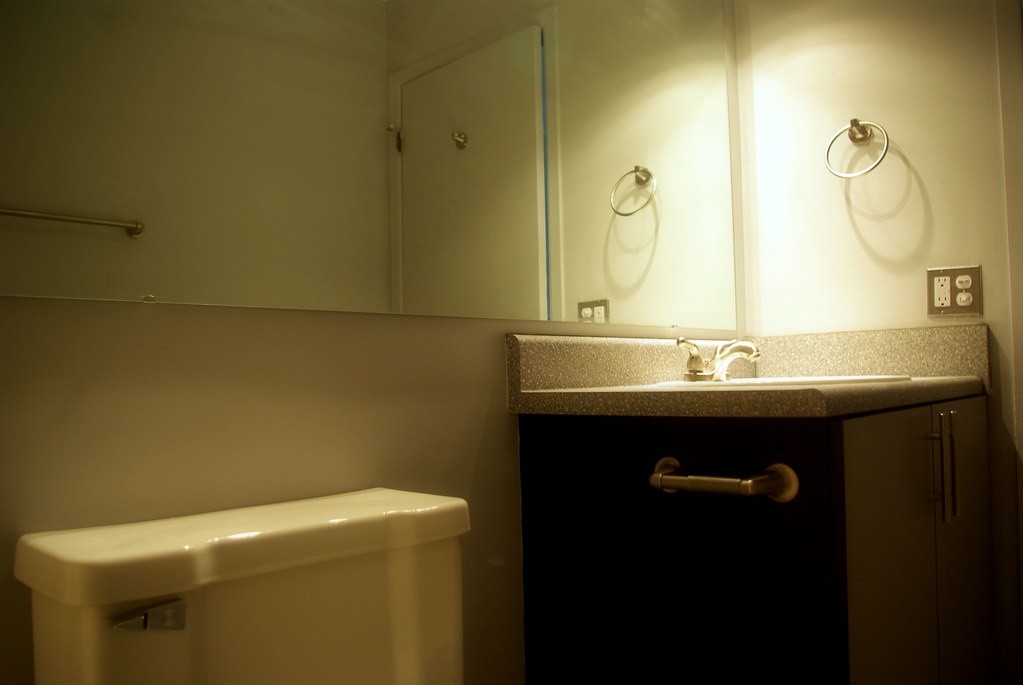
[0, 0, 734, 325]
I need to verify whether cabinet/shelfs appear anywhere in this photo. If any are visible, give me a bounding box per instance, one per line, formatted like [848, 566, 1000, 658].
[844, 394, 999, 685]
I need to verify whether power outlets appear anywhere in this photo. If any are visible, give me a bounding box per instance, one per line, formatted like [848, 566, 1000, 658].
[926, 264, 981, 316]
[576, 300, 610, 326]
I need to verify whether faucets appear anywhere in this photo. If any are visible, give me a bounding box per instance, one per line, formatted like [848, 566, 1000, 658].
[704, 338, 761, 382]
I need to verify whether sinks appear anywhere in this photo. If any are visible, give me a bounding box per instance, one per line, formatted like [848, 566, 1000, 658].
[658, 375, 911, 385]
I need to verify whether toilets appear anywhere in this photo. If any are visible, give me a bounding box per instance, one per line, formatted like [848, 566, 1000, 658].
[12, 486, 472, 684]
